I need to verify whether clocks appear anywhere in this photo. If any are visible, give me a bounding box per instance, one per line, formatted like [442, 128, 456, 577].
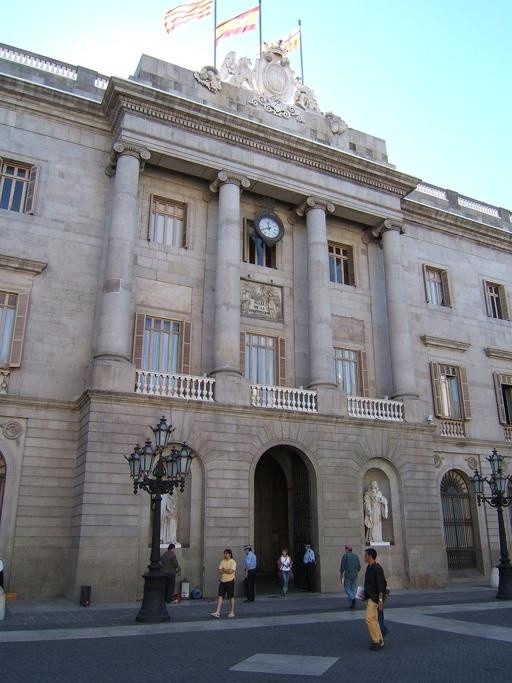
[253, 196, 285, 248]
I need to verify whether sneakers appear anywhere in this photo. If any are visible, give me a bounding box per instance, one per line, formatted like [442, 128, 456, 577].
[228, 613, 235, 618]
[370, 643, 383, 650]
[210, 612, 221, 618]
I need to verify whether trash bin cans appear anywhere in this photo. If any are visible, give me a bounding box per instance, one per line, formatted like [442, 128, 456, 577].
[181, 580, 189, 601]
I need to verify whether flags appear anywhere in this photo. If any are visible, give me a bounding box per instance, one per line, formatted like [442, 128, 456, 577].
[162, 0, 211, 33]
[212, 4, 259, 46]
[279, 28, 299, 53]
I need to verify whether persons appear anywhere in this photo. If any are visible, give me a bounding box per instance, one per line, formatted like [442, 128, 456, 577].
[210, 549, 236, 619]
[361, 543, 389, 650]
[160, 480, 178, 545]
[340, 543, 361, 609]
[302, 544, 317, 592]
[161, 544, 180, 604]
[365, 480, 390, 546]
[279, 548, 293, 597]
[242, 544, 258, 602]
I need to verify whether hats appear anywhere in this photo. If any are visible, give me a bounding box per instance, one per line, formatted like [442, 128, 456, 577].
[344, 543, 352, 549]
[243, 545, 251, 550]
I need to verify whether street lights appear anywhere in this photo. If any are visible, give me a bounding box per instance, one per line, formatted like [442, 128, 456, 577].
[467, 448, 512, 600]
[123, 415, 193, 623]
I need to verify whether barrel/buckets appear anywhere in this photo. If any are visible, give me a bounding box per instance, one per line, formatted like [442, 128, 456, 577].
[191, 588, 203, 600]
